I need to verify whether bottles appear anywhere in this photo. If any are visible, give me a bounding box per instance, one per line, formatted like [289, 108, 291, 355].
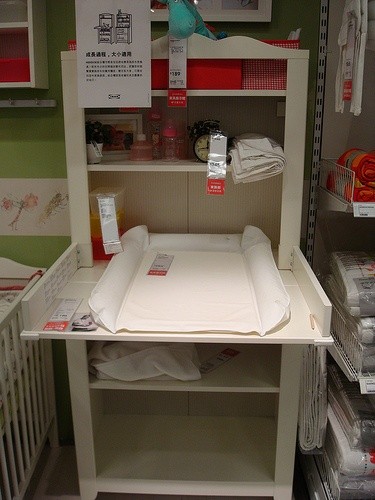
[148, 112, 164, 157]
[163, 120, 176, 160]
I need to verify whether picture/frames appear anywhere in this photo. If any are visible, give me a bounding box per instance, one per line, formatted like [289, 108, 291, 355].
[86, 114, 142, 155]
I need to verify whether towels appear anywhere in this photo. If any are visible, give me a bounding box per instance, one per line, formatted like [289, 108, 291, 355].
[322, 251, 375, 371]
[313, 402, 375, 500]
[228, 133, 287, 183]
[299, 345, 326, 451]
[326, 148, 375, 203]
[89, 341, 201, 381]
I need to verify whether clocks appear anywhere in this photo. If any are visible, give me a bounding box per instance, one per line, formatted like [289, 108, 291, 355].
[191, 118, 221, 162]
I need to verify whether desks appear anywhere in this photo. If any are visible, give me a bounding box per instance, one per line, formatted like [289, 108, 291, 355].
[18, 241, 337, 500]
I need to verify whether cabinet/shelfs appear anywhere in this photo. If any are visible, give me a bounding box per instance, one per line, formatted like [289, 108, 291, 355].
[306, 0, 375, 500]
[0, 0, 49, 89]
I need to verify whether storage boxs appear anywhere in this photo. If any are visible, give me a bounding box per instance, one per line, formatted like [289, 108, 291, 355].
[89, 186, 127, 260]
